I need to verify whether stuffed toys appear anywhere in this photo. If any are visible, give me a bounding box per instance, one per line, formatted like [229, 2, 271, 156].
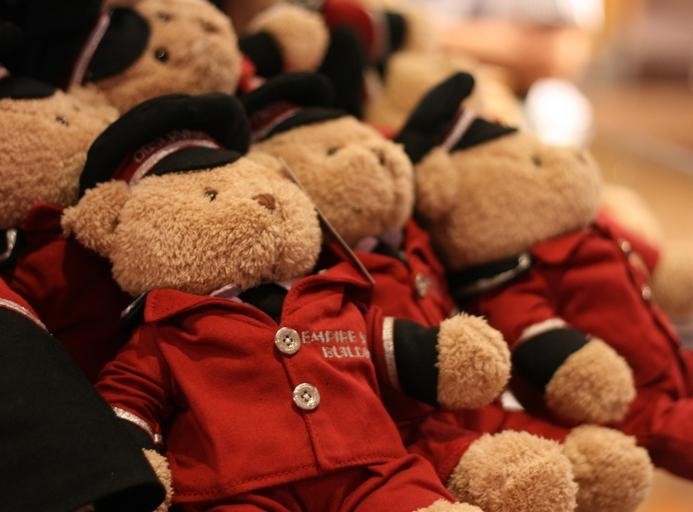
[0, 1, 692, 511]
